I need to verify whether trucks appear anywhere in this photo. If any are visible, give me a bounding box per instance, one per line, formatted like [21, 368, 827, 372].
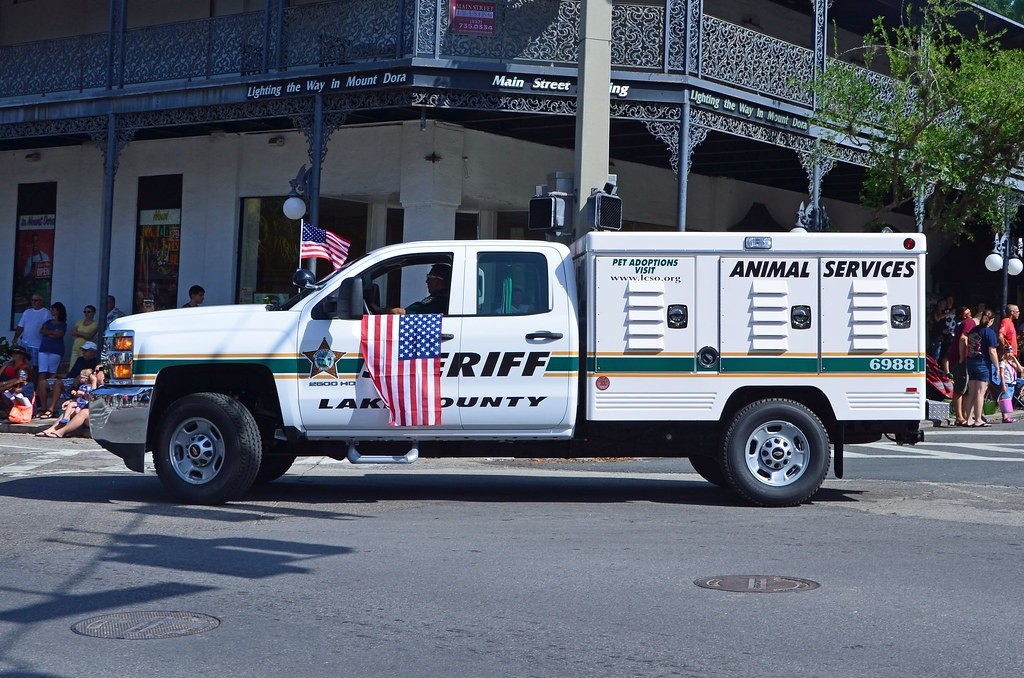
[88, 231, 951, 506]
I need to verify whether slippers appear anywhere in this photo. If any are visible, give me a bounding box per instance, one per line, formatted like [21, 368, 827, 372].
[36, 431, 64, 438]
[954, 416, 992, 427]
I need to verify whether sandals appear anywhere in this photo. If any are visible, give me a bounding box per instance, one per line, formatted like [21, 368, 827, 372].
[33, 409, 55, 419]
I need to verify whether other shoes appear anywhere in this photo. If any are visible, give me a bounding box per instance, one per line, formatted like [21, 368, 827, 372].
[1001, 416, 1016, 423]
[55, 371, 68, 379]
[59, 418, 69, 424]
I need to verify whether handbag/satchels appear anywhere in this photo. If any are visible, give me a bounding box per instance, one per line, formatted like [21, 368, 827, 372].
[8, 402, 33, 424]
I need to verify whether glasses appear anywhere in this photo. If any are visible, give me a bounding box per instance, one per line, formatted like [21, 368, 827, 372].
[31, 299, 41, 302]
[92, 369, 100, 374]
[84, 311, 94, 313]
[427, 274, 445, 281]
[82, 349, 90, 351]
[80, 374, 89, 379]
[12, 351, 23, 354]
[1010, 310, 1020, 313]
[18, 373, 28, 377]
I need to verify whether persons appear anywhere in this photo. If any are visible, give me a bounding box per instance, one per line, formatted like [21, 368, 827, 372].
[378, 262, 451, 316]
[107, 295, 126, 325]
[926, 296, 1023, 427]
[0, 294, 105, 439]
[183, 285, 206, 308]
[495, 287, 530, 313]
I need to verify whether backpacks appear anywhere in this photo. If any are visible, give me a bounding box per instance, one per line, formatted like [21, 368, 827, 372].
[956, 360, 969, 396]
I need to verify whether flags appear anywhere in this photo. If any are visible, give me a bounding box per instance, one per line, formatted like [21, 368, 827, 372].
[361, 314, 442, 427]
[301, 220, 351, 271]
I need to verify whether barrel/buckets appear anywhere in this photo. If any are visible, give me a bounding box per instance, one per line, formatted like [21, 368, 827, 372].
[983, 392, 996, 415]
[997, 391, 1014, 413]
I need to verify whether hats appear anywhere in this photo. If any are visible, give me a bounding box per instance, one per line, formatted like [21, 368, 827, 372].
[49, 304, 63, 308]
[9, 346, 31, 362]
[80, 341, 97, 349]
